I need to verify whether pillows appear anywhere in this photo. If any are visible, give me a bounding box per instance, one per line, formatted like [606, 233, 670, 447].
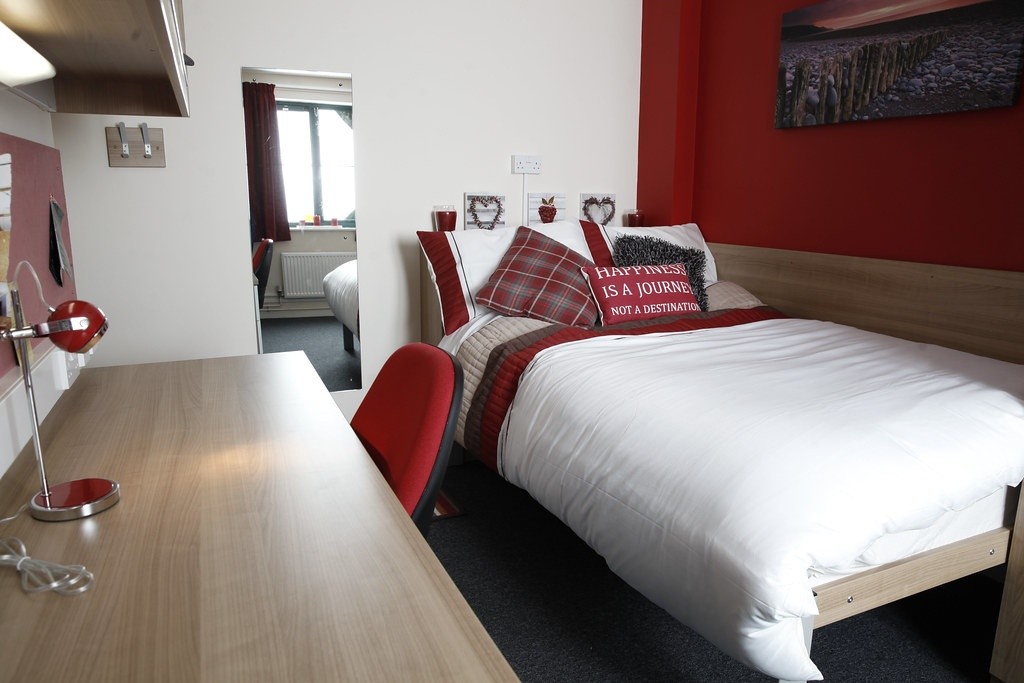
[615, 234, 712, 313]
[603, 223, 719, 291]
[474, 225, 598, 330]
[416, 220, 617, 337]
[580, 263, 701, 328]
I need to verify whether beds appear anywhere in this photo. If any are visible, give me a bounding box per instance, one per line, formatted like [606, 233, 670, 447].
[323, 259, 359, 353]
[418, 242, 1024, 683]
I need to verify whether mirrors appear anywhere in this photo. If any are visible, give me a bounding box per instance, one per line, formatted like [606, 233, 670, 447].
[241, 67, 362, 392]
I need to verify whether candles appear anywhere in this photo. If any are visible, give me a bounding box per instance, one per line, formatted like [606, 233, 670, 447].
[299, 220, 305, 226]
[331, 217, 338, 226]
[313, 215, 320, 225]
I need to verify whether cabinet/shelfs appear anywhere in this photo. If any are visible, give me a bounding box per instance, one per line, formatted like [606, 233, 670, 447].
[0, 0, 191, 118]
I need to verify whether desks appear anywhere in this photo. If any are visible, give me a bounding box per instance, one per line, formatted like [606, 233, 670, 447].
[0, 351, 520, 683]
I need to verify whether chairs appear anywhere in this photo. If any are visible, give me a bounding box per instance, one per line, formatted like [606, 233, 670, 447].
[350, 341, 465, 541]
[252, 239, 274, 310]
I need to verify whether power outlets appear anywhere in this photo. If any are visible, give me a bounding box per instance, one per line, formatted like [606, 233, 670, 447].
[511, 155, 542, 175]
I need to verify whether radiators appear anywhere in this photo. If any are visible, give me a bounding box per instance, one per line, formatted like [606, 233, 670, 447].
[280, 252, 356, 299]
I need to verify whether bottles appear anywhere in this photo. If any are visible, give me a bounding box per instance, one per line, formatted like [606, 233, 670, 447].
[435, 205, 457, 231]
[627, 209, 644, 226]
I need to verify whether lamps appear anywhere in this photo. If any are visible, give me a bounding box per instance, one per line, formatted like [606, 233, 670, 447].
[0, 260, 120, 522]
